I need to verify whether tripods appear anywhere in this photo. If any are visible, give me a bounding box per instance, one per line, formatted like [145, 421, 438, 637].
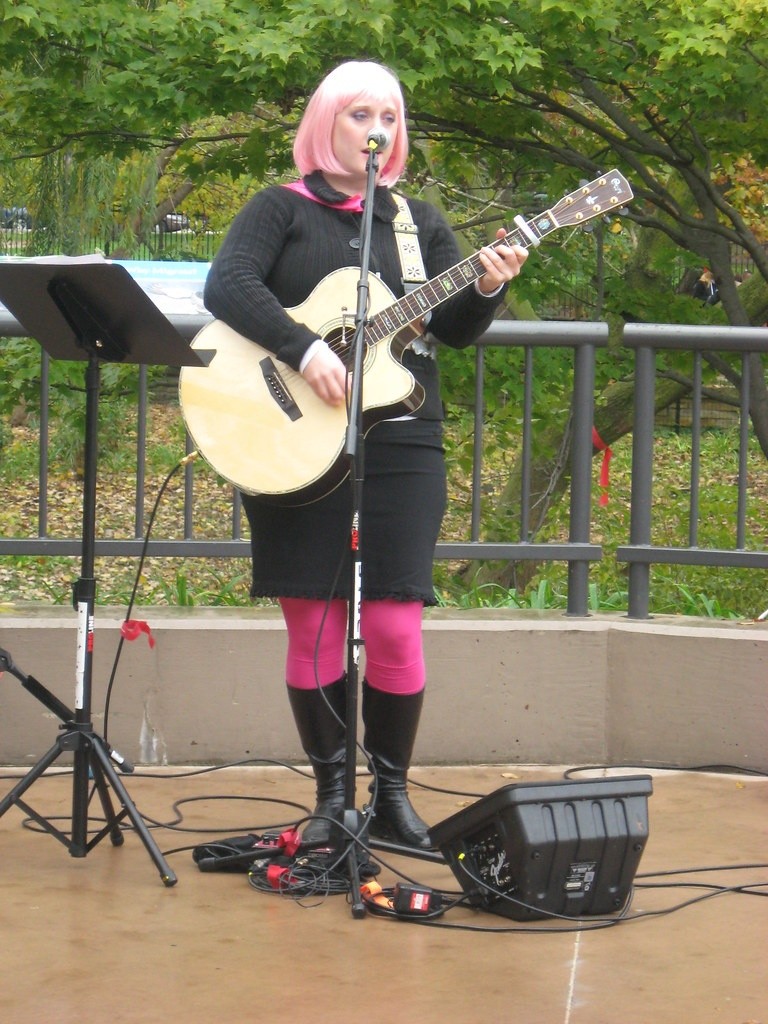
[0, 263, 216, 886]
[199, 150, 449, 920]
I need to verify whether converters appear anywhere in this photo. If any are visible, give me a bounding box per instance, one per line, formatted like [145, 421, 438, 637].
[392, 881, 443, 917]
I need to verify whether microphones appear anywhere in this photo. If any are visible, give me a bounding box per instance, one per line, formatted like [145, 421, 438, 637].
[368, 127, 391, 152]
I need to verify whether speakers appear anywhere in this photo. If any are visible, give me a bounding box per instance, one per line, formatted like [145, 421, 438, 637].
[426, 773, 652, 921]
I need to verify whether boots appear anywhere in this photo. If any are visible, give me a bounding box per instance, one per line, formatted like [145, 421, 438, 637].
[285, 672, 357, 854]
[362, 676, 443, 857]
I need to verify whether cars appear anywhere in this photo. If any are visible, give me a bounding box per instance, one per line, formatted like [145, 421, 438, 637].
[0, 206, 44, 231]
[156, 213, 189, 237]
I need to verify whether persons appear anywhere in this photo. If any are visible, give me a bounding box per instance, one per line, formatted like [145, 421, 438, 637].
[199, 60, 530, 854]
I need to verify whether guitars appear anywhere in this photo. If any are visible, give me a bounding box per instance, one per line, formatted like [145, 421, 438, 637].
[174, 162, 643, 505]
